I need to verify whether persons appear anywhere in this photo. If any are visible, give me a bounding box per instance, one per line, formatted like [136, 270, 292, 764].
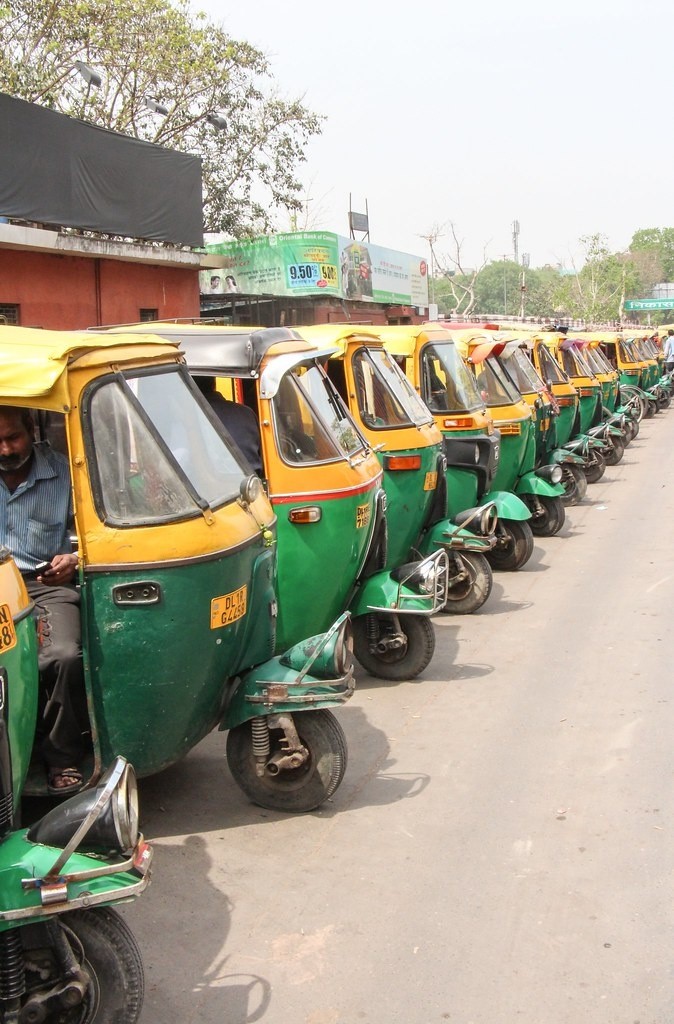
[477, 360, 488, 391]
[207, 276, 223, 294]
[193, 375, 262, 480]
[0, 405, 81, 795]
[224, 276, 241, 293]
[664, 329, 674, 372]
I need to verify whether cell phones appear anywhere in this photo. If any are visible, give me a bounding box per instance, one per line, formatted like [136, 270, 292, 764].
[35, 561, 56, 577]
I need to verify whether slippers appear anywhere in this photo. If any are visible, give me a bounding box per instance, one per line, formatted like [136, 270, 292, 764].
[46, 761, 84, 794]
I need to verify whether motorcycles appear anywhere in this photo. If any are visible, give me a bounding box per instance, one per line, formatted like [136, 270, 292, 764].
[2, 323, 673, 1024]
